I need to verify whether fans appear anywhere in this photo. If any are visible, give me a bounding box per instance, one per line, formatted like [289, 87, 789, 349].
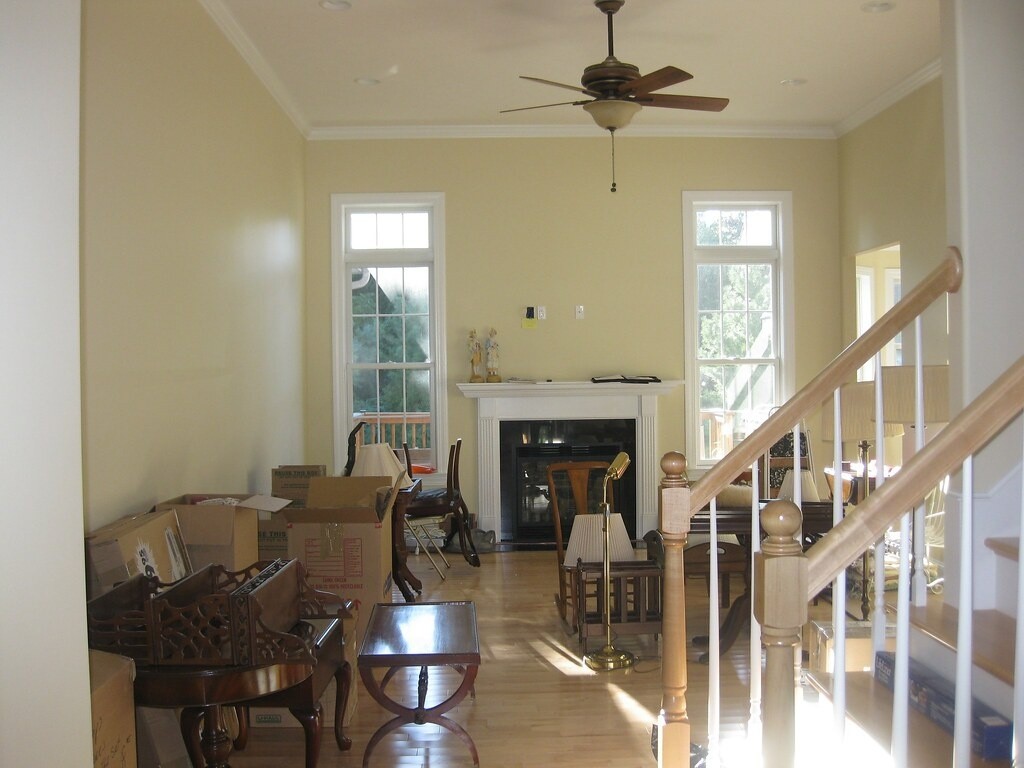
[495, 0, 731, 200]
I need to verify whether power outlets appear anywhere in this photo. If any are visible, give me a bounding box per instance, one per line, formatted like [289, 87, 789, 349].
[537, 306, 546, 320]
[575, 305, 584, 320]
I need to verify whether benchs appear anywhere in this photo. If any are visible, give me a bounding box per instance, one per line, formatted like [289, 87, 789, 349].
[87, 557, 351, 768]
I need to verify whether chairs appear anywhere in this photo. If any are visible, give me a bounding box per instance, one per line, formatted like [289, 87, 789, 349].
[339, 420, 481, 602]
[547, 460, 664, 657]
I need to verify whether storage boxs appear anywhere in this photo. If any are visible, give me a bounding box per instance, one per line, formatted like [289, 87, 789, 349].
[85, 460, 408, 727]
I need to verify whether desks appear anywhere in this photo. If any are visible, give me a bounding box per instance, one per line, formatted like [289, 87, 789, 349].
[356, 598, 484, 768]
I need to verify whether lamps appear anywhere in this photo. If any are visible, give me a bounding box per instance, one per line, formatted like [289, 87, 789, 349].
[777, 470, 820, 503]
[866, 362, 951, 607]
[820, 378, 905, 623]
